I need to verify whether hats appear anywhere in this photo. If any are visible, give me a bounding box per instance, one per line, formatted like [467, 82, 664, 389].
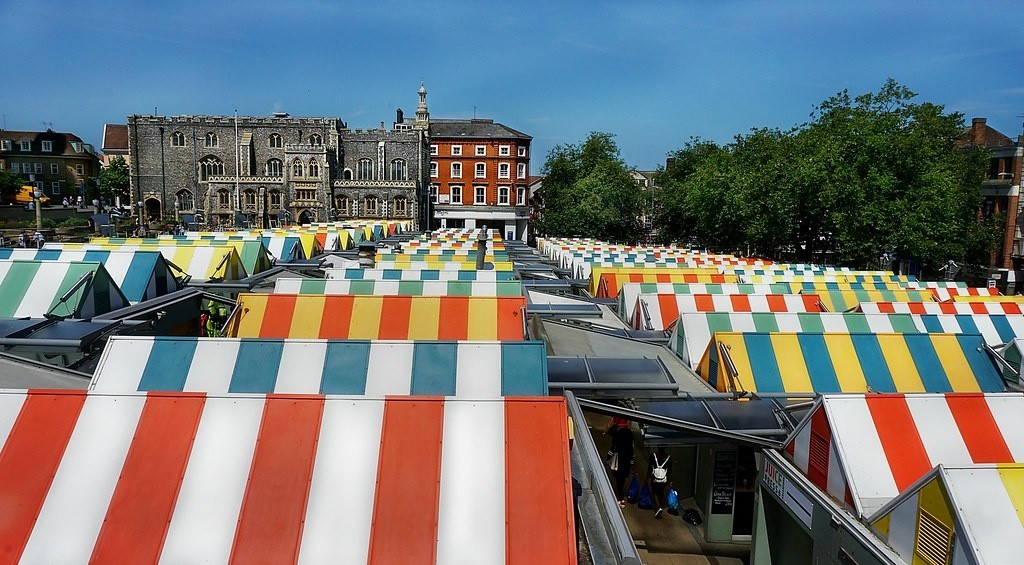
[618, 419, 630, 427]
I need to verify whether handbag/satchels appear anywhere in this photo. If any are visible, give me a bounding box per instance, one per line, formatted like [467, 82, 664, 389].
[668, 488, 680, 512]
[605, 452, 620, 471]
[638, 480, 652, 510]
[607, 423, 618, 438]
[627, 475, 640, 505]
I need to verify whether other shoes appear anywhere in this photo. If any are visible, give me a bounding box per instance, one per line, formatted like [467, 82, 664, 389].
[655, 508, 663, 518]
[618, 501, 626, 509]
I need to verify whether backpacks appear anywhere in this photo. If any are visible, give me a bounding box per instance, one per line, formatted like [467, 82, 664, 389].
[653, 452, 671, 484]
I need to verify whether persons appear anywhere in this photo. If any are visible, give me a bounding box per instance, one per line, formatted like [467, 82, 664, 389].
[18, 230, 29, 249]
[647, 442, 673, 519]
[62, 196, 69, 209]
[602, 416, 636, 508]
